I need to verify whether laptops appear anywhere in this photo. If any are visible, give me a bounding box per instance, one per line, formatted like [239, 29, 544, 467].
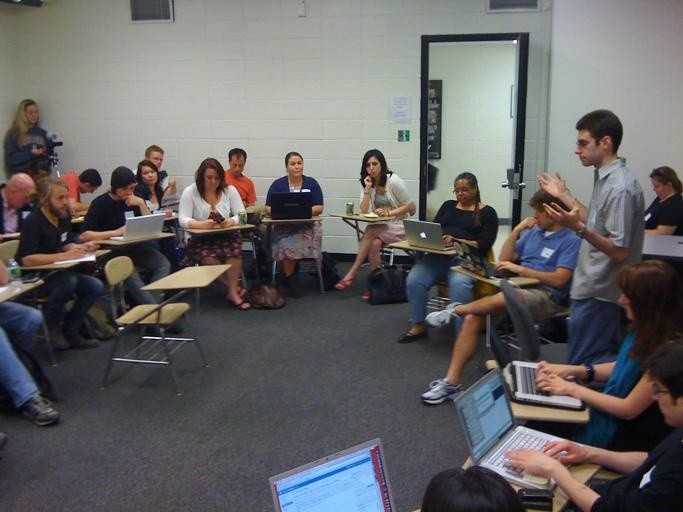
[403, 219, 455, 250]
[452, 239, 518, 279]
[271, 192, 311, 220]
[453, 368, 577, 490]
[268, 437, 396, 512]
[110, 214, 164, 241]
[484, 313, 586, 411]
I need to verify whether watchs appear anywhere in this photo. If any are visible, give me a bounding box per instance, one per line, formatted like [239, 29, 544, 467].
[572, 224, 589, 242]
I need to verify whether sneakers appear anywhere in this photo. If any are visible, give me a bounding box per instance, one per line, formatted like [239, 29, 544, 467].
[421, 378, 464, 404]
[22, 397, 58, 425]
[45, 317, 69, 349]
[399, 326, 428, 342]
[72, 337, 99, 348]
[425, 302, 464, 327]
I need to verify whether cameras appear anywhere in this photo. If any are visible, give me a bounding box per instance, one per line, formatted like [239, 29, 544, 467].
[41, 141, 63, 152]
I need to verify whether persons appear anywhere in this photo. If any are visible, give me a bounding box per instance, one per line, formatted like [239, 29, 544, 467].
[422, 259, 683, 512]
[642, 166, 683, 237]
[6, 98, 54, 176]
[178, 147, 258, 311]
[266, 152, 324, 288]
[336, 150, 416, 301]
[1, 145, 179, 424]
[423, 188, 580, 404]
[397, 173, 499, 343]
[539, 108, 645, 357]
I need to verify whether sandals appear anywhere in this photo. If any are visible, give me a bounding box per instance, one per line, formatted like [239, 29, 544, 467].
[335, 278, 353, 289]
[225, 285, 251, 310]
[361, 292, 370, 302]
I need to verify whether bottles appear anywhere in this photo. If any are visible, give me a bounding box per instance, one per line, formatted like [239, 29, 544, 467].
[6, 259, 23, 292]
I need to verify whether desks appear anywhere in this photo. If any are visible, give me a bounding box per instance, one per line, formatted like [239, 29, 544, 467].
[330, 209, 547, 293]
[139, 261, 233, 299]
[1, 276, 43, 310]
[17, 209, 323, 280]
[460, 354, 603, 509]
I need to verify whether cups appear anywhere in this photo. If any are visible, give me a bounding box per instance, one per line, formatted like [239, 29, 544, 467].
[164, 208, 172, 218]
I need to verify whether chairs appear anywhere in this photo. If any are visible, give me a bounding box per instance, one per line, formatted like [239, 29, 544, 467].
[0, 239, 90, 370]
[100, 254, 212, 396]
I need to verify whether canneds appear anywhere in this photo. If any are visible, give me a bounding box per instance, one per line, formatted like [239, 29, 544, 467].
[345, 202, 354, 216]
[239, 212, 245, 225]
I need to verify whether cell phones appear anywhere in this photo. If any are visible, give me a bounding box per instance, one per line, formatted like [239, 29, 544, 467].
[213, 218, 224, 223]
[23, 277, 40, 283]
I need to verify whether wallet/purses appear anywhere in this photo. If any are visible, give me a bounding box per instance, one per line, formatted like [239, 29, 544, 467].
[518, 489, 555, 511]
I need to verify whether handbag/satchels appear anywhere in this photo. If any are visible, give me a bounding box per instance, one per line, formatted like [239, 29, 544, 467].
[368, 264, 413, 305]
[246, 283, 286, 309]
[321, 251, 341, 291]
